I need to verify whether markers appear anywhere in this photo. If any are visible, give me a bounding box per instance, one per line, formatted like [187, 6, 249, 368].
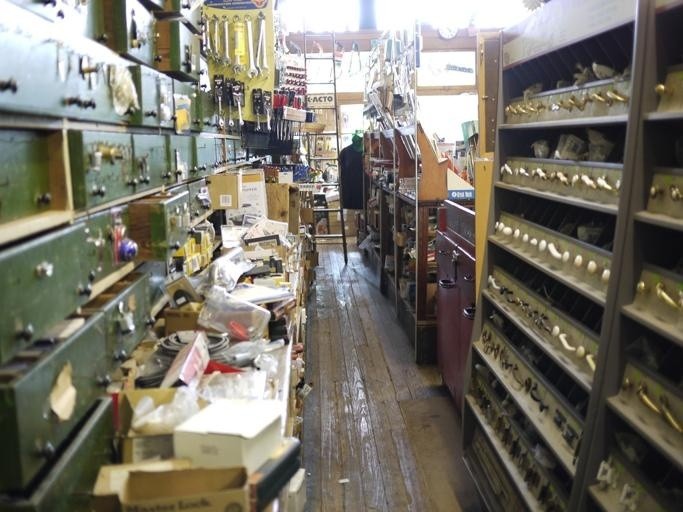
[263, 337, 289, 352]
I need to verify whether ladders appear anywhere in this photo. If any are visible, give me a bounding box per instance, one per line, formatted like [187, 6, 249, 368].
[303, 31, 347, 265]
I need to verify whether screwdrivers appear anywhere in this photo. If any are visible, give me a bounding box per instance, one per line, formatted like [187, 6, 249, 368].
[272, 90, 301, 140]
